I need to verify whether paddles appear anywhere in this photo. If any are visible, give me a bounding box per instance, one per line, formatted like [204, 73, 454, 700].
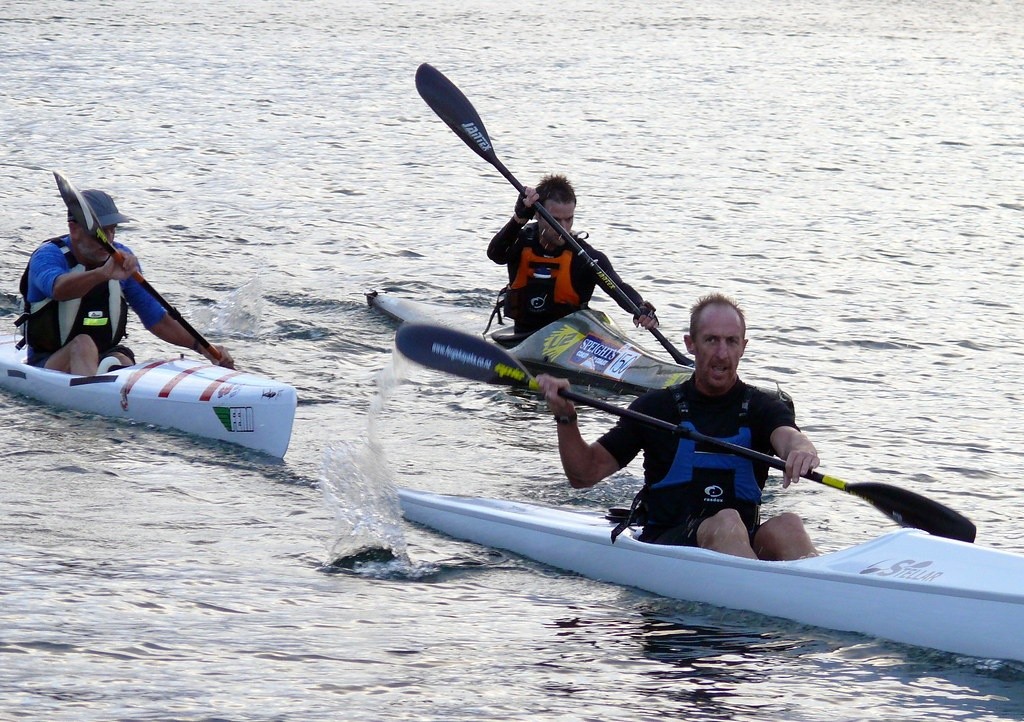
[53, 172, 238, 371]
[416, 62, 696, 369]
[395, 323, 977, 543]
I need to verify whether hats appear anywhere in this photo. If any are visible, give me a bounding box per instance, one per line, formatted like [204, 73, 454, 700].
[68, 190, 129, 227]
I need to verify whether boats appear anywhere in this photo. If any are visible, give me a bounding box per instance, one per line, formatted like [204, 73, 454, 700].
[1, 332, 294, 458]
[367, 292, 695, 395]
[395, 487, 1024, 664]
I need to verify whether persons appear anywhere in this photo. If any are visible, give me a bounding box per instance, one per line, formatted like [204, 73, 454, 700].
[485, 175, 659, 346]
[532, 294, 821, 563]
[20, 188, 234, 378]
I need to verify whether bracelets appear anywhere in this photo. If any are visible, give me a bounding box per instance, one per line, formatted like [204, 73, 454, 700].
[193, 339, 199, 350]
[554, 412, 577, 424]
